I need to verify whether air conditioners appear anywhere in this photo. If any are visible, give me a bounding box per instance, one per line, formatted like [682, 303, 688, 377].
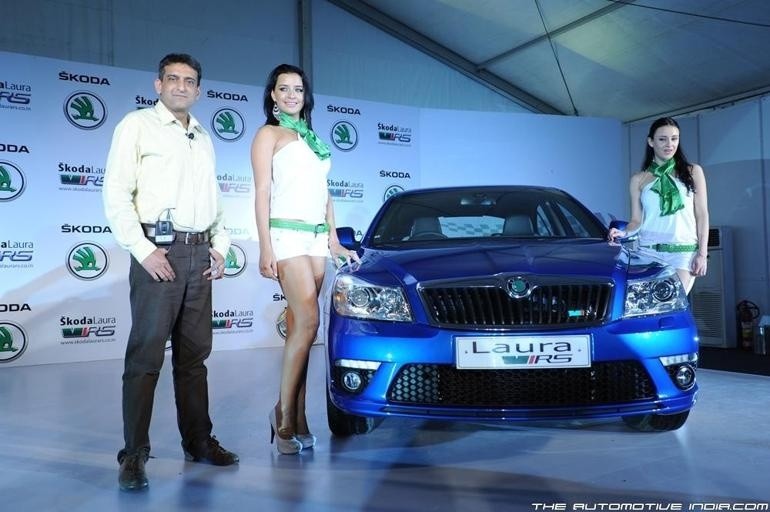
[690, 224, 737, 348]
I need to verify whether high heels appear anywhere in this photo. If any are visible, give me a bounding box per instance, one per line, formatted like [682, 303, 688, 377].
[296, 432, 316, 450]
[268, 406, 304, 455]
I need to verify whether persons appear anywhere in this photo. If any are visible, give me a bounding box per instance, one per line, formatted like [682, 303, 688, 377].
[249, 60, 363, 457]
[606, 116, 711, 301]
[100, 52, 242, 497]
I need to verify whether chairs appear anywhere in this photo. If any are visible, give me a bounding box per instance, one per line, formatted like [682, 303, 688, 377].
[503, 214, 534, 234]
[410, 216, 442, 237]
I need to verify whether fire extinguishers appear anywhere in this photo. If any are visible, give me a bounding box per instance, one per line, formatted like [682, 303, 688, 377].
[737, 300, 760, 349]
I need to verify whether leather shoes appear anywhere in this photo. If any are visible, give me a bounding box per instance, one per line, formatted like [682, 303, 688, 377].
[118, 447, 157, 494]
[183, 434, 239, 466]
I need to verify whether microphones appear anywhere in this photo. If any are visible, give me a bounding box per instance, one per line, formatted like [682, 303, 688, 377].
[188, 133, 195, 139]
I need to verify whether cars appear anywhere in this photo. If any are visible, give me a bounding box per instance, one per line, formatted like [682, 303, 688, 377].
[320, 179, 707, 439]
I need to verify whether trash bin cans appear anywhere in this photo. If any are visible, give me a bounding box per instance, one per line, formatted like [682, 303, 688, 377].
[753, 326, 770, 355]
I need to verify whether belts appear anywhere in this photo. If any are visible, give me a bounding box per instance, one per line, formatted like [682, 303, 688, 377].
[642, 243, 698, 254]
[269, 220, 330, 237]
[145, 225, 211, 246]
